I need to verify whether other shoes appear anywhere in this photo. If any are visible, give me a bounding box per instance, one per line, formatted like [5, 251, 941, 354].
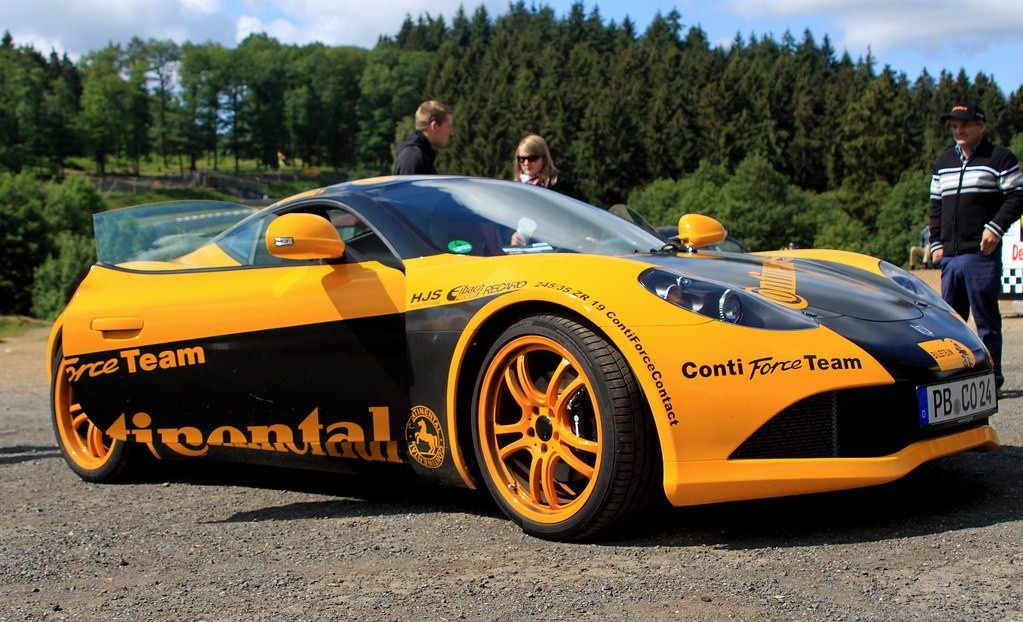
[922, 263, 928, 270]
[909, 265, 915, 270]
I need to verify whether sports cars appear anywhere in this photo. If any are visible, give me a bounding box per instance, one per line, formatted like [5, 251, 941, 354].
[43, 174, 1000, 544]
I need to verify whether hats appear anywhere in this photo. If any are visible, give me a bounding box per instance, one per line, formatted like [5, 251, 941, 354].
[941, 103, 985, 124]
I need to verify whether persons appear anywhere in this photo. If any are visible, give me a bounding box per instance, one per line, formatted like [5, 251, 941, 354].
[497, 134, 574, 246]
[910, 219, 931, 270]
[393, 100, 453, 175]
[929, 104, 1023, 398]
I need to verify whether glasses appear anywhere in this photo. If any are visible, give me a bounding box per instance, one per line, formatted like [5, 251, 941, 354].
[516, 155, 542, 162]
